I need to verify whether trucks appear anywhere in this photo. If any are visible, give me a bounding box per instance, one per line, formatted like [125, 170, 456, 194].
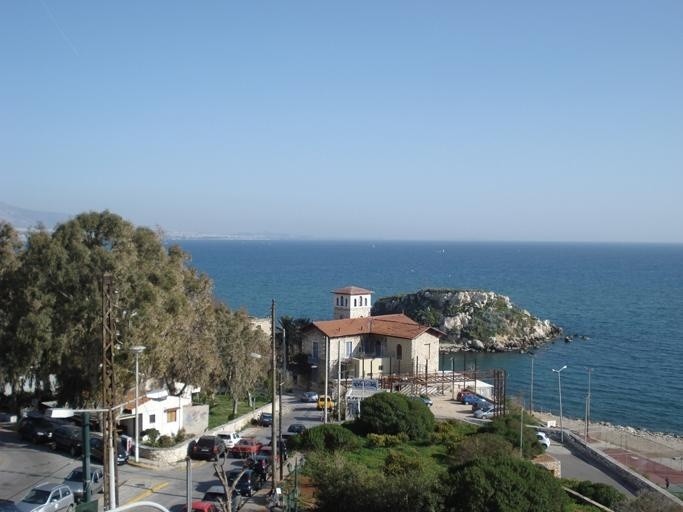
[316, 394, 333, 411]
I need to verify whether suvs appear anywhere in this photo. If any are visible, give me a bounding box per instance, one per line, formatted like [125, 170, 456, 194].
[14, 482, 75, 512]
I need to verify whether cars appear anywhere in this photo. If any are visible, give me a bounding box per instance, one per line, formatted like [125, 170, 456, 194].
[192, 434, 227, 462]
[88, 431, 130, 466]
[178, 468, 262, 512]
[53, 424, 83, 457]
[217, 430, 242, 452]
[16, 416, 55, 445]
[258, 412, 273, 426]
[242, 423, 306, 482]
[300, 391, 319, 403]
[457, 391, 509, 419]
[535, 431, 551, 450]
[231, 438, 263, 458]
[61, 465, 104, 502]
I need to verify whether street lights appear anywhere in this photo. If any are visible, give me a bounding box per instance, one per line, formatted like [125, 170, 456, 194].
[128, 345, 148, 464]
[43, 386, 169, 510]
[552, 365, 568, 443]
